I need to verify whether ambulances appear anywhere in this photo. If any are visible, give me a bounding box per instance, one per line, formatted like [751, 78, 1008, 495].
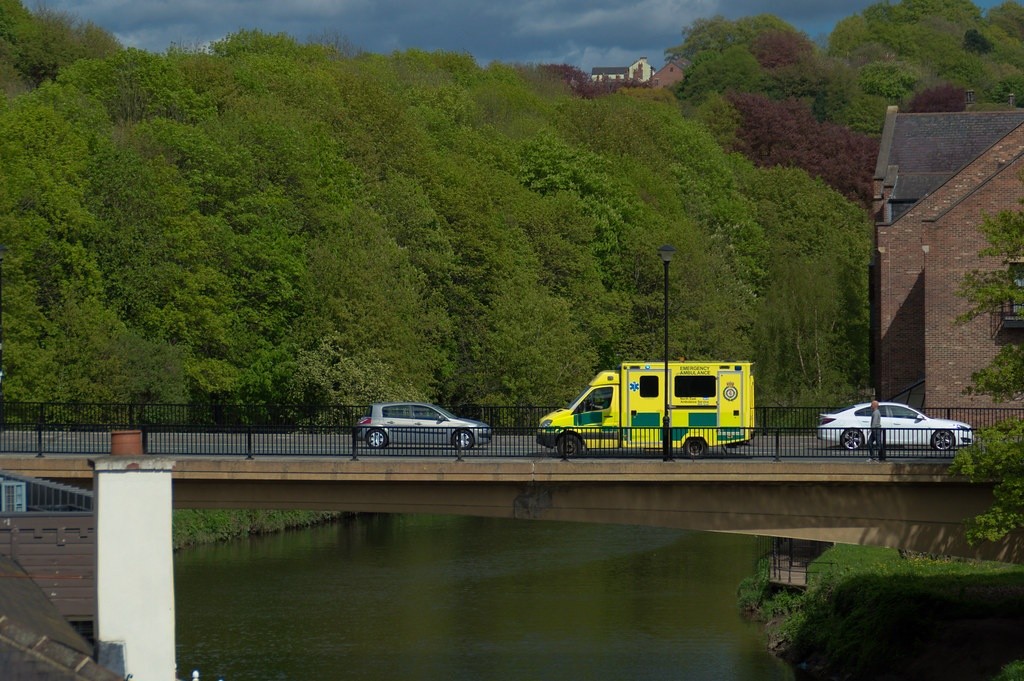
[537, 358, 755, 458]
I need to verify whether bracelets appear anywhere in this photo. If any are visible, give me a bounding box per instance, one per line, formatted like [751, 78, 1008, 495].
[594, 404, 595, 406]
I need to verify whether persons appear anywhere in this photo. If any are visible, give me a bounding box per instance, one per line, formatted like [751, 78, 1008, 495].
[588, 389, 611, 409]
[867, 401, 882, 462]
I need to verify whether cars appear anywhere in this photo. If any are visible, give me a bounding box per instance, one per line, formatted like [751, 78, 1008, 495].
[817, 402, 975, 451]
[353, 403, 491, 450]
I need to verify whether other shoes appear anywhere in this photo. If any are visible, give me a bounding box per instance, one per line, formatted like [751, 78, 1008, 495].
[866, 458, 878, 462]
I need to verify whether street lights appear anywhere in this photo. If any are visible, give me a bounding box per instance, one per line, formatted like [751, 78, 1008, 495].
[659, 245, 675, 461]
